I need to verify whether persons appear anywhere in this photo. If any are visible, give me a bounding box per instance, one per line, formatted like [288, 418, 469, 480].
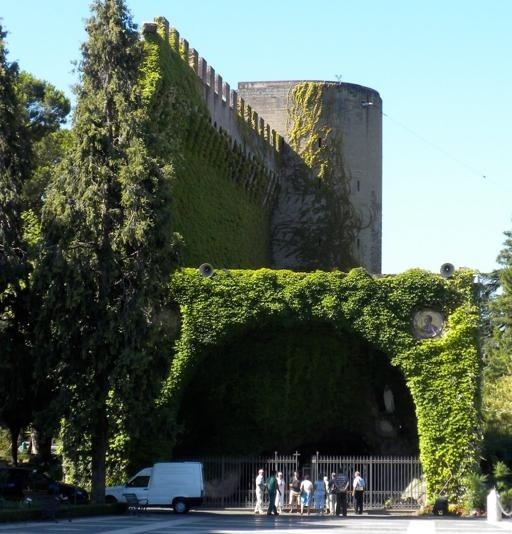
[255, 468, 365, 517]
[26, 469, 68, 519]
[420, 314, 438, 333]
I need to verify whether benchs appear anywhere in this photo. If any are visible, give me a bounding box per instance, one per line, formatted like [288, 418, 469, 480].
[34, 490, 72, 523]
[122, 493, 148, 516]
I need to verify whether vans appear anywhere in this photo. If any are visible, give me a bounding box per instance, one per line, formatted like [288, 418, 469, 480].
[0, 466, 90, 506]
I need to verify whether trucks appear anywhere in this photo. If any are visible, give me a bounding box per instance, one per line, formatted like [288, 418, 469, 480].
[105, 462, 205, 513]
